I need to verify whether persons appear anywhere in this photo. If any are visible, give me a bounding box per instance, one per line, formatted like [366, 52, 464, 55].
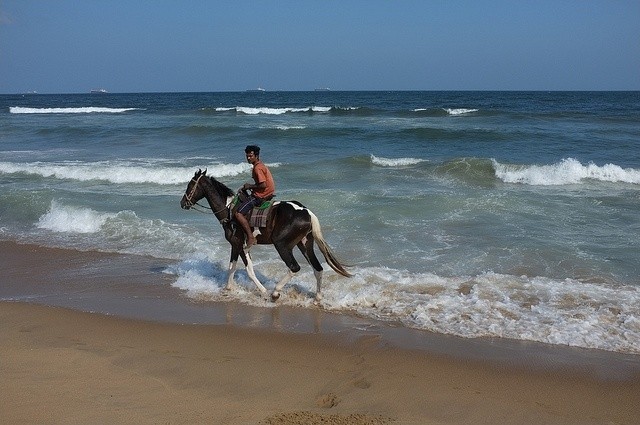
[235, 145, 275, 249]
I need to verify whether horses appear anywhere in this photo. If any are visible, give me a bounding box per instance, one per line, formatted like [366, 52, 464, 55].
[179, 168, 358, 305]
